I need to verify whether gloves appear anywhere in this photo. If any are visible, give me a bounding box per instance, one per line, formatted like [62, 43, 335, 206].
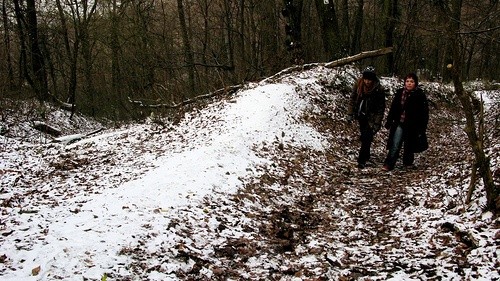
[385, 121, 390, 130]
[371, 124, 380, 134]
[347, 115, 353, 124]
[416, 134, 422, 139]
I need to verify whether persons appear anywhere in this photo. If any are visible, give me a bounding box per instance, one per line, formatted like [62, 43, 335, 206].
[346, 67, 386, 169]
[382, 73, 430, 170]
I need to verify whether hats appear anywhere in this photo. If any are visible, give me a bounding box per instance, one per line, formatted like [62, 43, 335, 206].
[363, 66, 377, 79]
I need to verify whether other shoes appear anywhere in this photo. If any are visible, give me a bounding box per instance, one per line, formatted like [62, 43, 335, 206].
[406, 165, 413, 168]
[358, 161, 366, 168]
[382, 165, 390, 171]
